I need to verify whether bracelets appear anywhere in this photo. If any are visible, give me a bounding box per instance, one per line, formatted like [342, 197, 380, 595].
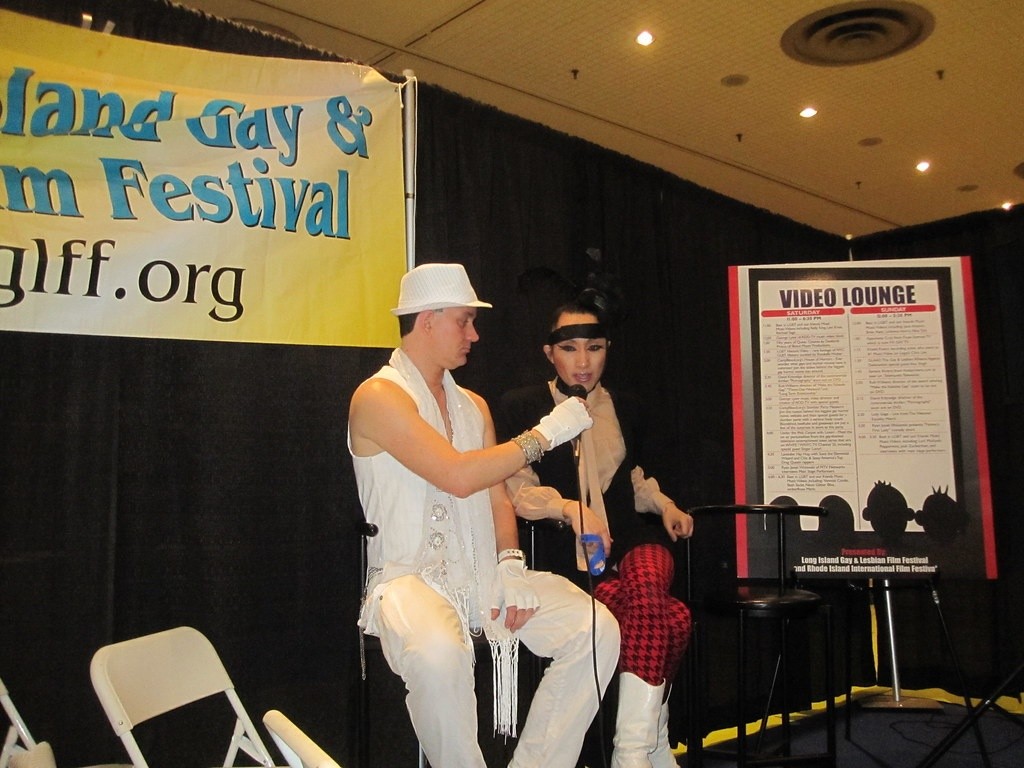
[498, 549, 524, 563]
[511, 430, 545, 468]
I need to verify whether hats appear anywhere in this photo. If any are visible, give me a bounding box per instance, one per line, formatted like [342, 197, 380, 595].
[389, 263, 493, 316]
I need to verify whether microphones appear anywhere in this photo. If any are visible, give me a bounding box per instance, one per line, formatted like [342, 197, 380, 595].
[569, 384, 588, 465]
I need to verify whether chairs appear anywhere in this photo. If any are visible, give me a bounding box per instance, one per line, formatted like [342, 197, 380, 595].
[0, 515, 541, 768]
[683, 501, 835, 768]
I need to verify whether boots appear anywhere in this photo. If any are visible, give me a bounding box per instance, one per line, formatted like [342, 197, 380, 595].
[613, 672, 666, 768]
[652, 685, 681, 767]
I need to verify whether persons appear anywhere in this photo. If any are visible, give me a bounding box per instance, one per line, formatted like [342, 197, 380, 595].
[347, 263, 621, 768]
[493, 301, 693, 768]
